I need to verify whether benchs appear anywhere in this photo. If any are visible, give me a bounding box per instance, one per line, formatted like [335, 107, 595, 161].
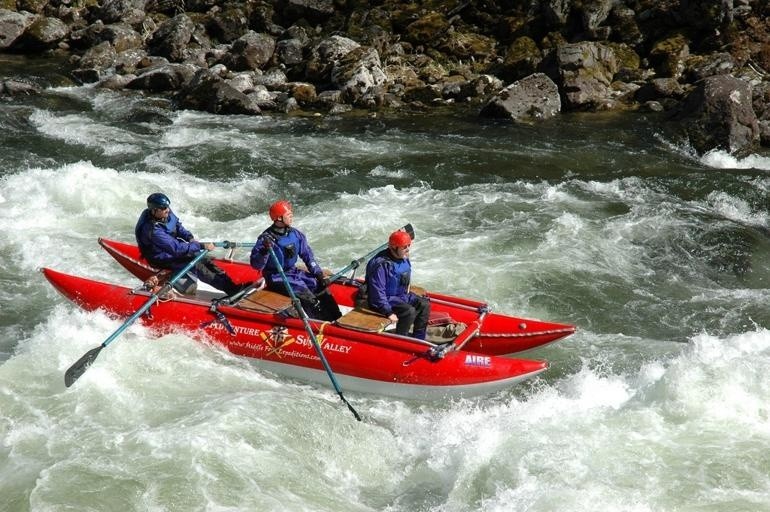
[237, 267, 332, 314]
[336, 285, 425, 333]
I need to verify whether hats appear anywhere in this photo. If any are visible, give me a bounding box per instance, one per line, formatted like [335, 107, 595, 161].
[268, 201, 292, 220]
[146, 193, 171, 211]
[388, 231, 412, 250]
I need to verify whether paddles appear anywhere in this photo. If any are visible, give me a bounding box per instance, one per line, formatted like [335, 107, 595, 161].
[65, 242, 215, 389]
[279, 223, 414, 320]
[267, 246, 363, 425]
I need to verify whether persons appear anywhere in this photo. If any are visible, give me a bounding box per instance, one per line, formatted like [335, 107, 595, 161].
[366, 231, 430, 341]
[248, 201, 342, 321]
[134, 195, 255, 297]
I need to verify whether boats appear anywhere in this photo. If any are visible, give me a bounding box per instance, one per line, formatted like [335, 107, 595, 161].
[38, 237, 577, 402]
